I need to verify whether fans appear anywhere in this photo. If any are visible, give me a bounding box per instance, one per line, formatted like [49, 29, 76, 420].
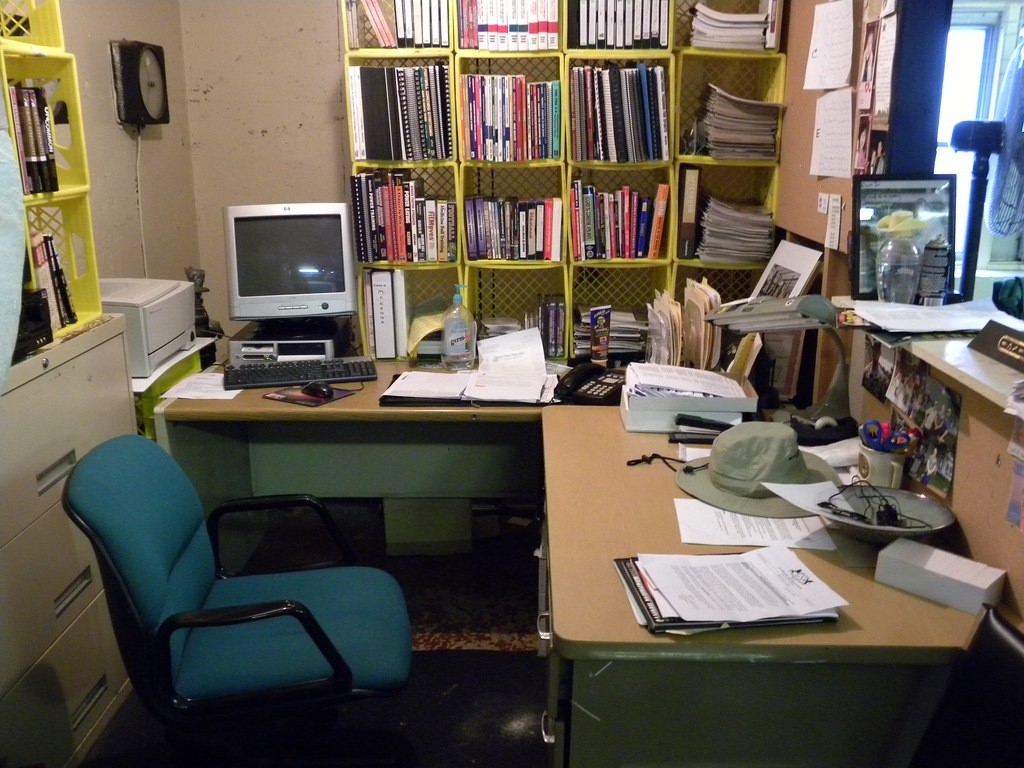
[950, 61, 1024, 303]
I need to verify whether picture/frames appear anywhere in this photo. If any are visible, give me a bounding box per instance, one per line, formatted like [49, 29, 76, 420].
[852, 174, 956, 302]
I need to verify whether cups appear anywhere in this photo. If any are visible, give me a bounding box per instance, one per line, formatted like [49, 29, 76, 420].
[857, 440, 906, 489]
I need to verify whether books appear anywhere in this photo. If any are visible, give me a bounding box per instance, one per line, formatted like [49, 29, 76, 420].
[9, 85, 60, 196]
[378, 370, 557, 408]
[613, 541, 850, 634]
[31, 231, 77, 334]
[346, 0, 783, 360]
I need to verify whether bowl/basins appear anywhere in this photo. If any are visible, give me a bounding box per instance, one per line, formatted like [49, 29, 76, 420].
[836, 485, 955, 543]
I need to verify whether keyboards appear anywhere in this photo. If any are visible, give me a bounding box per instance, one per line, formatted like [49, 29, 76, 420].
[223, 356, 378, 390]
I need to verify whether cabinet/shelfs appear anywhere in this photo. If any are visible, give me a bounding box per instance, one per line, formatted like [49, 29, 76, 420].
[0, 0, 103, 342]
[337, 0, 786, 359]
[0, 312, 134, 768]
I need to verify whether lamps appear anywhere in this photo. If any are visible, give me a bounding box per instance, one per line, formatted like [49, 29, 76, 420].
[702, 294, 858, 447]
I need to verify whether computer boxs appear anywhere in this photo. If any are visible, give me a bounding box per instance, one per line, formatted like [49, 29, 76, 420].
[228, 317, 350, 366]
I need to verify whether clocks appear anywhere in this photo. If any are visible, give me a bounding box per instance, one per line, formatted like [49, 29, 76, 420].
[109, 39, 170, 129]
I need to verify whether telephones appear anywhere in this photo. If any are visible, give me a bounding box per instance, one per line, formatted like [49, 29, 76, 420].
[554, 361, 625, 406]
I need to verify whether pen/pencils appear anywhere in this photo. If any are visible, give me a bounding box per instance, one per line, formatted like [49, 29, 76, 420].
[882, 420, 921, 451]
[858, 425, 868, 446]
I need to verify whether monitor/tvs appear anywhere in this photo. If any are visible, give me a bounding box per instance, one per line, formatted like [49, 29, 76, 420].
[222, 203, 358, 342]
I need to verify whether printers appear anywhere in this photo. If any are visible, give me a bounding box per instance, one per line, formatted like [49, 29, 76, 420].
[100, 277, 197, 377]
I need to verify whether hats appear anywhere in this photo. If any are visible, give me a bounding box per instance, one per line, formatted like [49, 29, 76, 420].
[675, 421, 844, 519]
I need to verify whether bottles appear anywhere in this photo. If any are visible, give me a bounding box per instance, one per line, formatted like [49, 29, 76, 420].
[921, 234, 951, 307]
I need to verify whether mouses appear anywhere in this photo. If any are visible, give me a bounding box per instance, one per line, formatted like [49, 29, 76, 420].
[301, 381, 334, 398]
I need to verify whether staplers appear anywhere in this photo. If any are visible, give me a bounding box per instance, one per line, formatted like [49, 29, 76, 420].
[667, 413, 737, 443]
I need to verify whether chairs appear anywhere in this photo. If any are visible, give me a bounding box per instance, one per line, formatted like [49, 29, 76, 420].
[62, 434, 418, 768]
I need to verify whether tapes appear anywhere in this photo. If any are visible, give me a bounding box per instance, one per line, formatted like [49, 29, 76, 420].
[792, 415, 838, 430]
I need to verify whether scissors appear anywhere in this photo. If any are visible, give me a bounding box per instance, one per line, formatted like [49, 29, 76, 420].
[863, 419, 911, 453]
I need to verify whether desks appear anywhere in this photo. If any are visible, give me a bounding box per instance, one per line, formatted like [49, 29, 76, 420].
[132, 336, 204, 441]
[157, 355, 980, 768]
